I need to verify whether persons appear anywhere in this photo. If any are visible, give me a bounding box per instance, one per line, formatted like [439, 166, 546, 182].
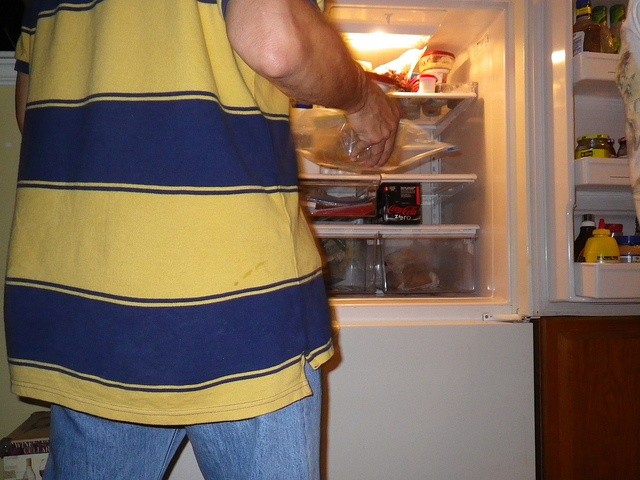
[2, 1, 401, 480]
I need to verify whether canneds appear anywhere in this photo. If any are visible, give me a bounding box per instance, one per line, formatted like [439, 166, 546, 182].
[614, 236, 640, 263]
[617, 137, 627, 157]
[575, 134, 617, 158]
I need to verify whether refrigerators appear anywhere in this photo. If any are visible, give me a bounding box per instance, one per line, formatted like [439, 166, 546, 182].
[168, 1, 634, 476]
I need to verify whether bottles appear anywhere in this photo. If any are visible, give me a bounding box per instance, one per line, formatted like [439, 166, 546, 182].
[572, 211, 597, 262]
[580, 227, 621, 264]
